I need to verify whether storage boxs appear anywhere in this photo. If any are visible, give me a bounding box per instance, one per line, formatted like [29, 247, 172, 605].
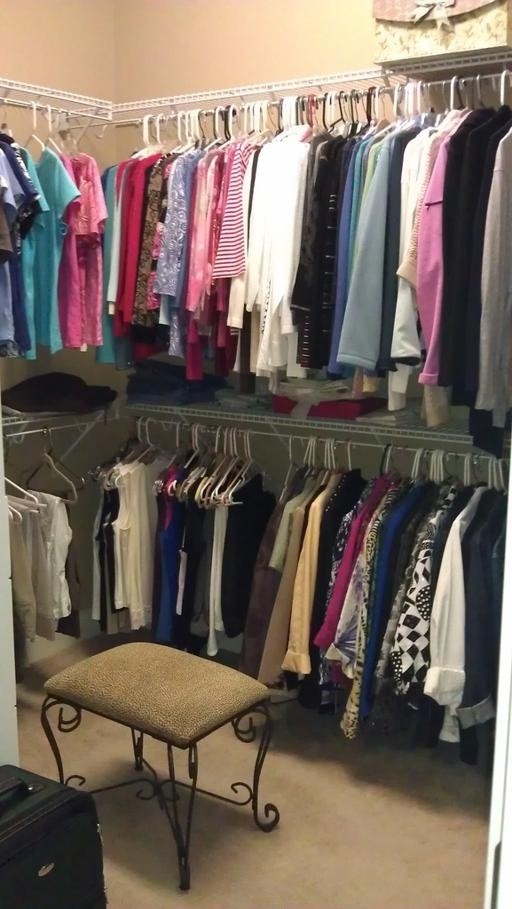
[371, 0, 510, 67]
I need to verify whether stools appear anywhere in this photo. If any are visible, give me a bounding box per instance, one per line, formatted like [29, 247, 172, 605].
[38, 639, 282, 892]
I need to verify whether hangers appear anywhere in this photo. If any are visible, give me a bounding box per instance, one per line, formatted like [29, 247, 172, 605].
[5, 430, 90, 522]
[0, 93, 110, 156]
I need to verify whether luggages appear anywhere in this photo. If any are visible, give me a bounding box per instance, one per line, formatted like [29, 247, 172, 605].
[0, 760, 108, 909]
